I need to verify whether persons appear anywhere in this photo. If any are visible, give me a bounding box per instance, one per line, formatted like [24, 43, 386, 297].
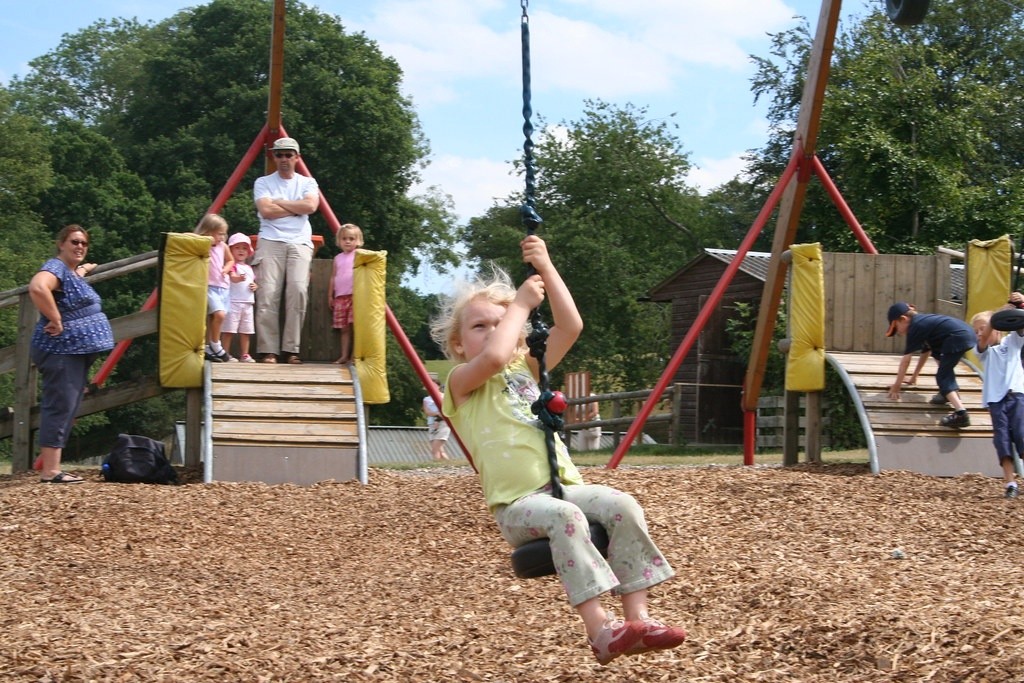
[423, 379, 451, 461]
[328, 224, 364, 362]
[253, 138, 320, 364]
[430, 234, 685, 666]
[970, 291, 1024, 499]
[220, 233, 258, 363]
[195, 214, 234, 362]
[575, 393, 601, 451]
[885, 302, 976, 427]
[28, 225, 114, 484]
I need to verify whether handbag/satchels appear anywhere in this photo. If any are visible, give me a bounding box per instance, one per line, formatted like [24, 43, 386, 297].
[98, 433, 178, 484]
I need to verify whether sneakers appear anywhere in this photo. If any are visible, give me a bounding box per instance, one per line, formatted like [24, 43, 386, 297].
[932, 390, 960, 405]
[940, 411, 971, 427]
[240, 354, 255, 362]
[228, 353, 239, 362]
[1005, 485, 1019, 500]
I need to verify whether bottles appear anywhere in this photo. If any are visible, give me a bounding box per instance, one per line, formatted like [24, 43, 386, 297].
[102, 464, 112, 480]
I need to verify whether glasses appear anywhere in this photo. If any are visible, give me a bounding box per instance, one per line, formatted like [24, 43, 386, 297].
[65, 239, 89, 247]
[275, 153, 295, 158]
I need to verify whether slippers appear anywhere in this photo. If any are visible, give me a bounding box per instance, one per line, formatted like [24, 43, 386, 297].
[40, 473, 86, 483]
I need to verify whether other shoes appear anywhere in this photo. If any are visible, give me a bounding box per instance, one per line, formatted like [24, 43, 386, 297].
[262, 353, 278, 363]
[333, 357, 349, 364]
[209, 342, 229, 362]
[287, 355, 303, 364]
[204, 351, 222, 362]
[625, 610, 685, 656]
[587, 611, 647, 665]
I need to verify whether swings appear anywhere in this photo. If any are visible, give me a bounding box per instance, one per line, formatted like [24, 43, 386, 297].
[508, 0, 613, 580]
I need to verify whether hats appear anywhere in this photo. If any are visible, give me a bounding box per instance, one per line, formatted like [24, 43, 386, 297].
[269, 138, 301, 155]
[885, 303, 910, 337]
[228, 233, 254, 257]
